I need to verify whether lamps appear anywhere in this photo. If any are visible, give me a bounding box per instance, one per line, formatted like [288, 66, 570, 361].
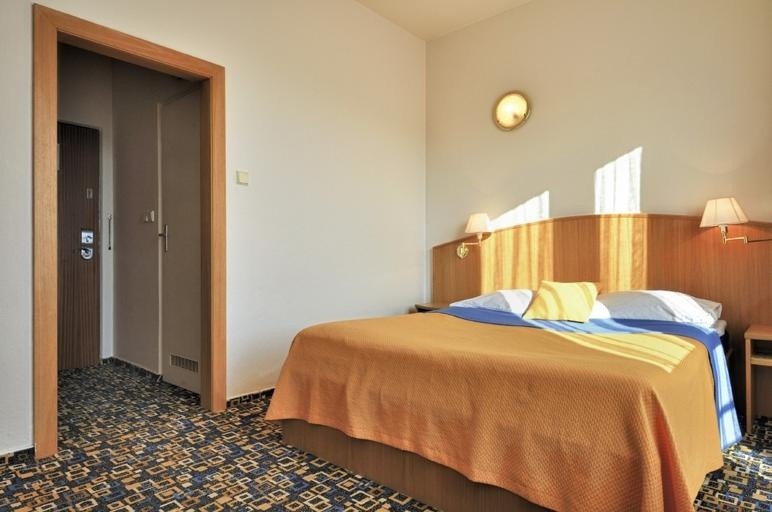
[455, 212, 495, 261]
[697, 194, 753, 245]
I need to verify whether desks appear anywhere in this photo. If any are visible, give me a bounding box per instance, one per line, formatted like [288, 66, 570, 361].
[740, 322, 772, 435]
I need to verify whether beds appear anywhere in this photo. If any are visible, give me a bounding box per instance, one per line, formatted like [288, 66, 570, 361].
[280, 210, 772, 512]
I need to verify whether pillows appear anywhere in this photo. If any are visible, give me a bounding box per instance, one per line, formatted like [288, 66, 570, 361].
[450, 276, 722, 332]
